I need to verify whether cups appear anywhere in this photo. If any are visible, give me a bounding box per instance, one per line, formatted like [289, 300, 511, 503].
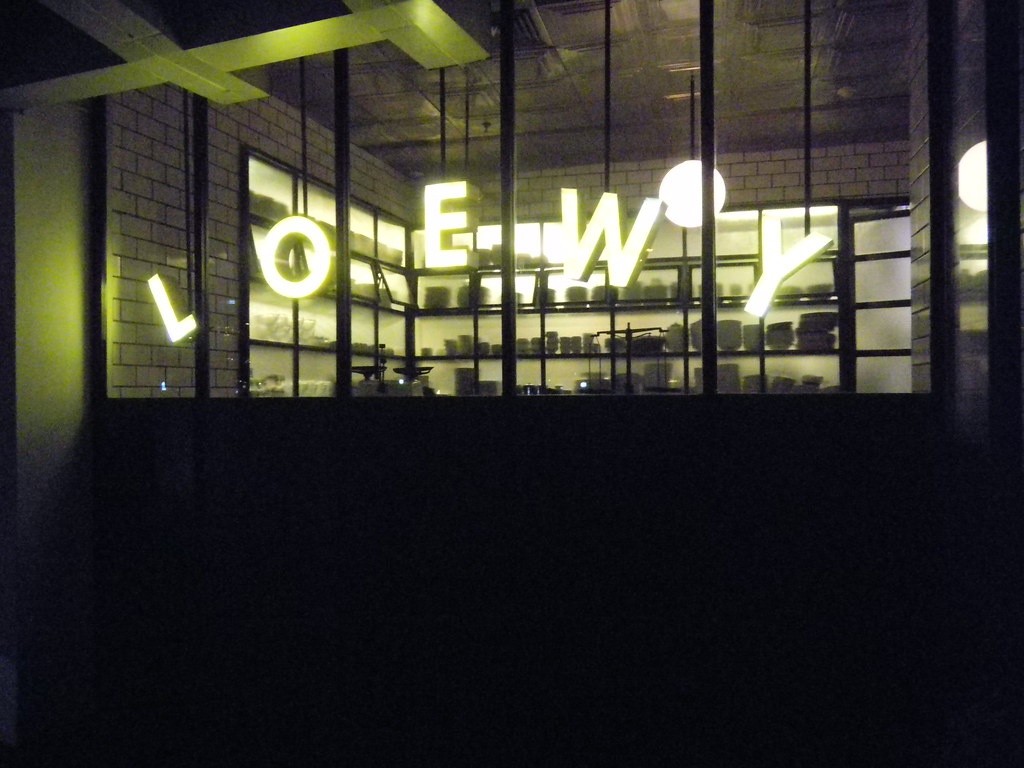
[283, 381, 331, 396]
[253, 312, 317, 345]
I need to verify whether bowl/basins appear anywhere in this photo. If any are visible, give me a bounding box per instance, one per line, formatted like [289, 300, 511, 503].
[424, 243, 838, 394]
